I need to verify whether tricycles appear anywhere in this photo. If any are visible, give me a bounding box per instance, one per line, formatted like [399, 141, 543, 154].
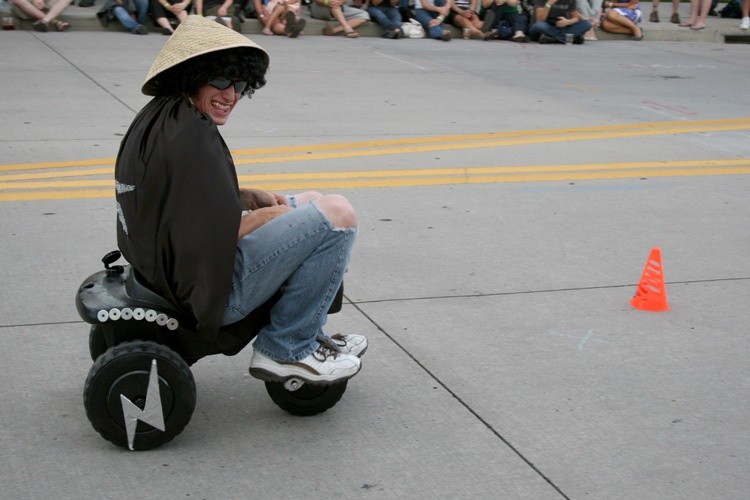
[73, 248, 349, 451]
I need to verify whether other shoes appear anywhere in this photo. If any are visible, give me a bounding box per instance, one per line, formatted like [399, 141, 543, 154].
[634, 27, 643, 41]
[691, 22, 706, 30]
[679, 22, 694, 27]
[650, 11, 658, 23]
[25, 22, 597, 45]
[671, 13, 680, 24]
[741, 16, 750, 29]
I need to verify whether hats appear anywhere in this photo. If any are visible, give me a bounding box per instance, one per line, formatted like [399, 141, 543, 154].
[141, 15, 270, 97]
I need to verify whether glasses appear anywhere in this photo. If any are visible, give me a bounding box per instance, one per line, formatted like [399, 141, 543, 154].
[199, 74, 250, 92]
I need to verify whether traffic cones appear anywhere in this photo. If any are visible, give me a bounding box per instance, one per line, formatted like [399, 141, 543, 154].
[631, 247, 670, 312]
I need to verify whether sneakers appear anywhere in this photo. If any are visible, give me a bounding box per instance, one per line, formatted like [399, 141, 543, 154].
[319, 333, 368, 356]
[248, 342, 363, 384]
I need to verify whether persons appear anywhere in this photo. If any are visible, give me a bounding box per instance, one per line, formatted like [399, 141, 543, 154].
[114, 14, 370, 386]
[10, 1, 74, 31]
[103, 0, 749, 48]
